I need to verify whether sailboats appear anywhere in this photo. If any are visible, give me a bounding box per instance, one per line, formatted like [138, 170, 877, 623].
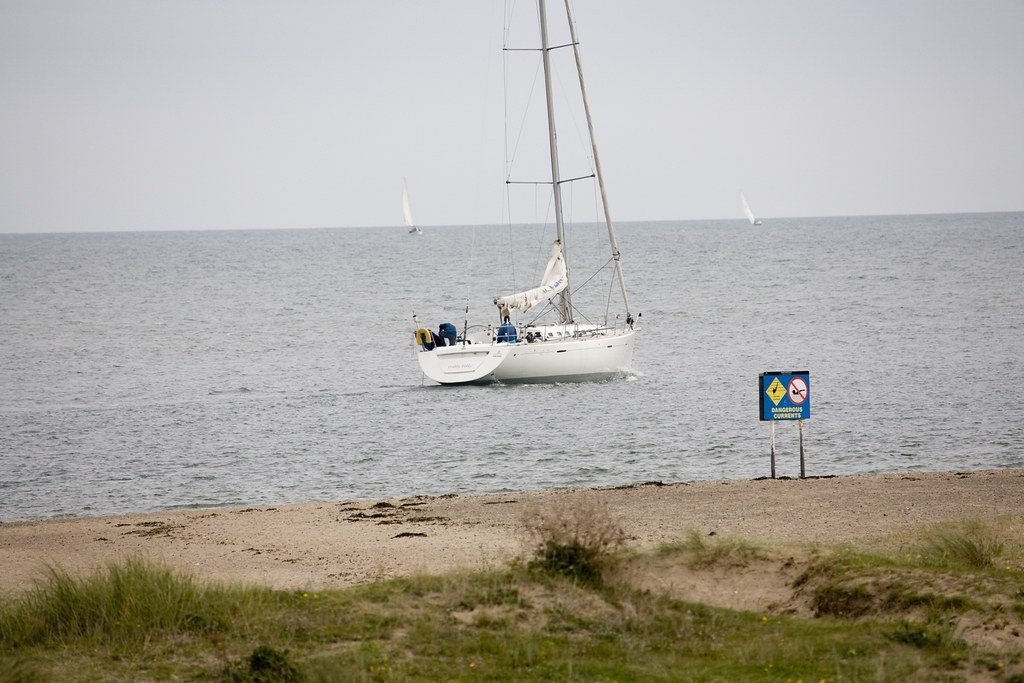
[411, 0, 643, 386]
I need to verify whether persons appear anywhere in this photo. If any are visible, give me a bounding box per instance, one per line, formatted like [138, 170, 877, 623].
[497, 315, 517, 344]
[439, 323, 456, 345]
[526, 332, 543, 343]
